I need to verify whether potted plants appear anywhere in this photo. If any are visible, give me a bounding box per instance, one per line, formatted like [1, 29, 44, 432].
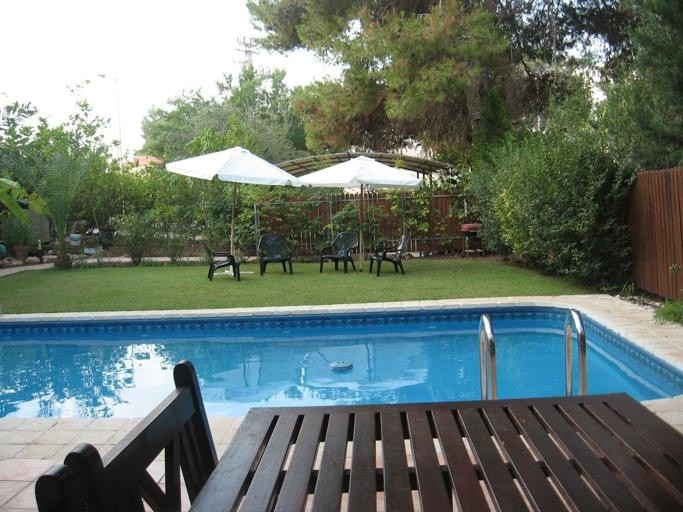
[12, 220, 32, 261]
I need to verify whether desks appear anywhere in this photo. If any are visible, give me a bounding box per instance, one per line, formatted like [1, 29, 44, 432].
[186, 393, 683, 511]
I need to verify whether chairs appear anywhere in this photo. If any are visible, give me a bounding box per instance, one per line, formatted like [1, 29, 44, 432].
[28, 359, 218, 512]
[200, 230, 411, 283]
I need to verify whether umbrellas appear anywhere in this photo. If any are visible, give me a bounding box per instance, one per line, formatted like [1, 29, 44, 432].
[298, 155, 422, 269]
[166, 145, 302, 256]
